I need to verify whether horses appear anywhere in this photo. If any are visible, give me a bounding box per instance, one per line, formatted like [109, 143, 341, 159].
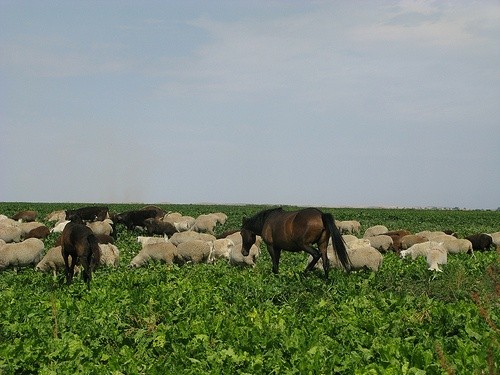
[65, 203, 111, 223]
[240, 205, 354, 285]
[111, 208, 162, 241]
[56, 222, 101, 289]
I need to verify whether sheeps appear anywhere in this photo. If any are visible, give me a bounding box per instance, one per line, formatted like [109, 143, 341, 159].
[211, 237, 236, 264]
[136, 233, 168, 248]
[0, 222, 23, 243]
[96, 242, 120, 270]
[306, 220, 500, 273]
[177, 239, 214, 265]
[48, 219, 71, 233]
[17, 219, 45, 233]
[19, 226, 50, 243]
[224, 233, 264, 255]
[34, 245, 85, 278]
[9, 208, 37, 222]
[48, 214, 67, 221]
[163, 210, 228, 234]
[127, 242, 177, 268]
[0, 238, 45, 270]
[230, 242, 260, 270]
[168, 231, 217, 247]
[85, 219, 114, 236]
[0, 214, 20, 225]
[44, 209, 66, 220]
